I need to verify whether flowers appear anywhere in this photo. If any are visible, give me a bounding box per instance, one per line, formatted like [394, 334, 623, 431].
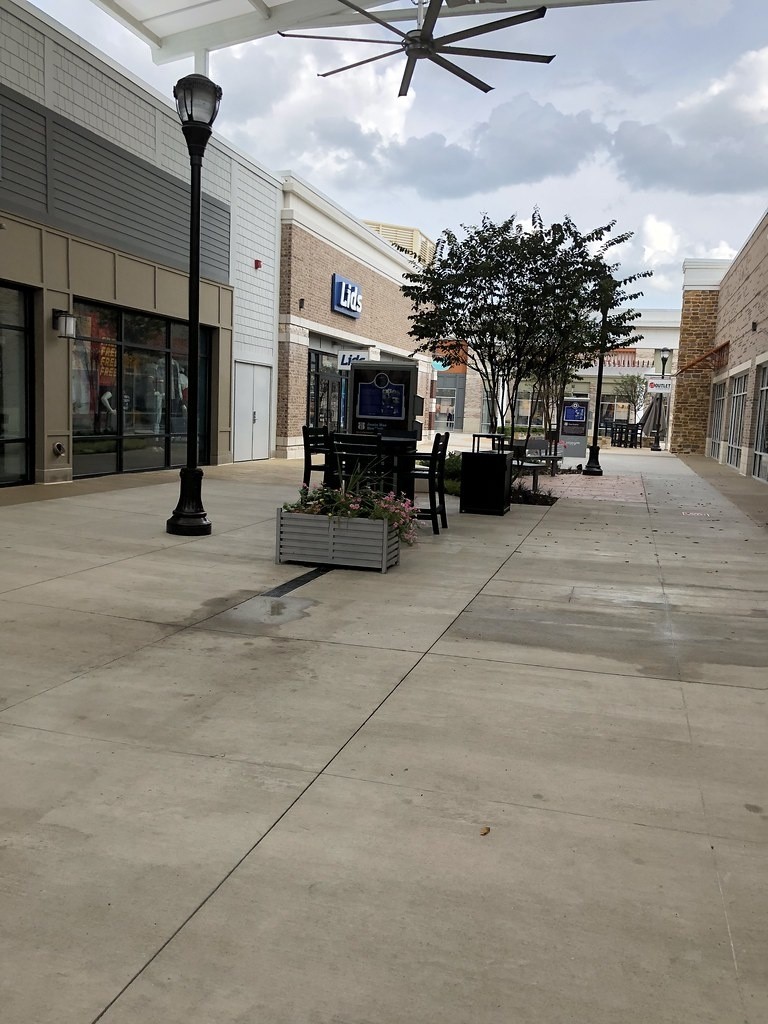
[282, 455, 427, 548]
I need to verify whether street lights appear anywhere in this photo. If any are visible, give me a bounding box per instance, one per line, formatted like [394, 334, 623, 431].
[167, 73, 222, 537]
[583, 273, 614, 474]
[651, 347, 672, 450]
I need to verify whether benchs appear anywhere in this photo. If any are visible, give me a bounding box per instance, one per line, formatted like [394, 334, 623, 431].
[513, 460, 546, 492]
[508, 439, 563, 477]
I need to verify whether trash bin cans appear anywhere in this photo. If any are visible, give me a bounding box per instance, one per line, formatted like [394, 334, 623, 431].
[459, 450, 514, 517]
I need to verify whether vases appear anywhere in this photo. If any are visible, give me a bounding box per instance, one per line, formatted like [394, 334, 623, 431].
[276, 507, 401, 573]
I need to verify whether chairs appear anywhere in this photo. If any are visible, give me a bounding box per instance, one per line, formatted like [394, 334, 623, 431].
[329, 430, 386, 494]
[605, 420, 644, 449]
[301, 425, 351, 503]
[400, 431, 452, 534]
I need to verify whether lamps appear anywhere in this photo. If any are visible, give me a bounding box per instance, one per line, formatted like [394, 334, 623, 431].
[52, 307, 77, 340]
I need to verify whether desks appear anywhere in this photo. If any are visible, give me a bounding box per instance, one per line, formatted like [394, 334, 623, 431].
[333, 436, 416, 507]
[612, 423, 637, 448]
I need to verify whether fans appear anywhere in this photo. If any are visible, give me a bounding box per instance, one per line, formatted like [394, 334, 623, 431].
[278, 0, 556, 98]
